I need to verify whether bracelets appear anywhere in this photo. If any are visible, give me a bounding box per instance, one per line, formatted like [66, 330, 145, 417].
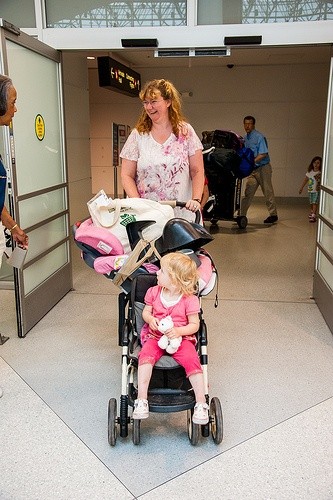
[11, 224, 17, 230]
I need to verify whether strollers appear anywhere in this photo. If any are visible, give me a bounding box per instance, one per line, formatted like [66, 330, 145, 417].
[108, 200, 224, 447]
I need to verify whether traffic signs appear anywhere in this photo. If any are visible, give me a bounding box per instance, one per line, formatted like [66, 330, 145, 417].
[97, 56, 141, 97]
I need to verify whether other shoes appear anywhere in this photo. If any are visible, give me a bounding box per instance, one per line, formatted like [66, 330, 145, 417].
[132, 398, 149, 420]
[308, 212, 317, 222]
[192, 402, 210, 425]
[264, 216, 278, 223]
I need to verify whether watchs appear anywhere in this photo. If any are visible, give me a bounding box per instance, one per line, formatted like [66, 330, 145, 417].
[194, 199, 201, 204]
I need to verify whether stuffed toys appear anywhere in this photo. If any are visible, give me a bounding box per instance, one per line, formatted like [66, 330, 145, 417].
[158, 316, 182, 354]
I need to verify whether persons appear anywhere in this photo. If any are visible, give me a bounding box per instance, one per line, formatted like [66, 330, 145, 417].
[0, 76, 31, 249]
[299, 157, 322, 222]
[119, 79, 204, 227]
[239, 116, 278, 224]
[133, 253, 210, 424]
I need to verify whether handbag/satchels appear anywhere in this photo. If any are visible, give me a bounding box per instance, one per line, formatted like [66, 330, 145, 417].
[87, 197, 175, 252]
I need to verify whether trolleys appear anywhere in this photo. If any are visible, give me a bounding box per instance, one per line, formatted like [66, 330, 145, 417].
[203, 162, 247, 230]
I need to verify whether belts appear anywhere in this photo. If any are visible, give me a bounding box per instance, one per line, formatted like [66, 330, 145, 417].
[254, 162, 269, 170]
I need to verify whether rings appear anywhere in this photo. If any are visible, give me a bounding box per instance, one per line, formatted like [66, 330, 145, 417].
[194, 206, 196, 209]
